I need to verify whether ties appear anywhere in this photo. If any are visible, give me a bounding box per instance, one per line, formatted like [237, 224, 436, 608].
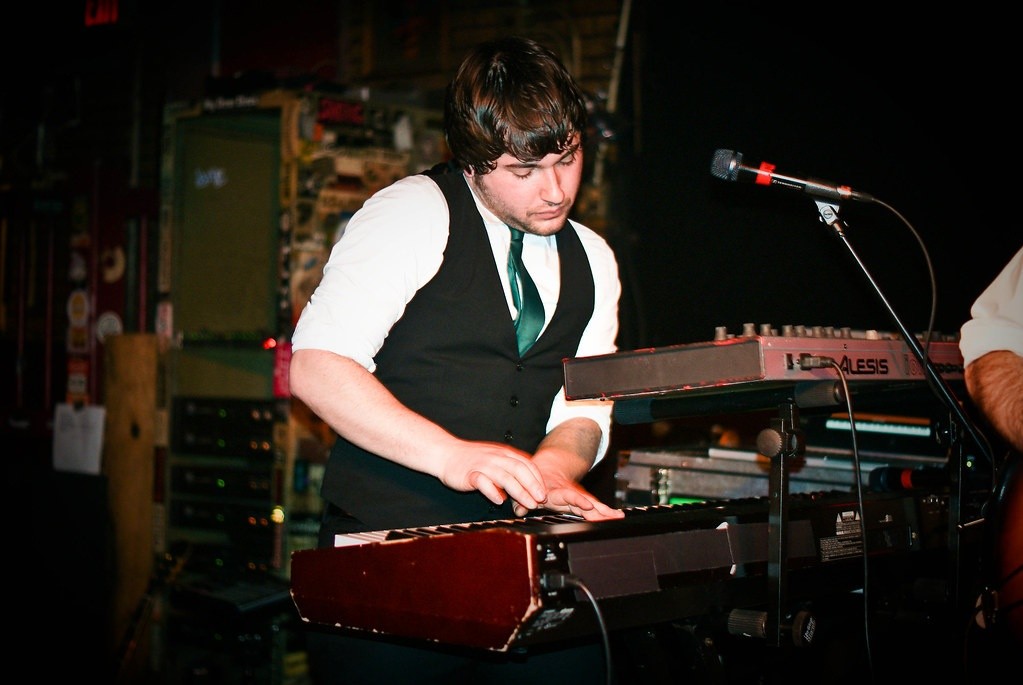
[507, 228, 546, 359]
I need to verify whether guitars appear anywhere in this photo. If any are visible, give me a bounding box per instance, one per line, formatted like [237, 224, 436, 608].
[987, 448, 1021, 639]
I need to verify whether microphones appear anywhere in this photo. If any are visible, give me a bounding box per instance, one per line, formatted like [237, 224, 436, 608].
[710, 149, 873, 209]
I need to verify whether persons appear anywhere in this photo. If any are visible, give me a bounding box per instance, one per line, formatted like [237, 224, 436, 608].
[958, 245, 1023, 449]
[289, 35, 627, 685]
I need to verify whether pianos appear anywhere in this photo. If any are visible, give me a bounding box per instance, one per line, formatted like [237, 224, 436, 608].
[284, 481, 948, 656]
